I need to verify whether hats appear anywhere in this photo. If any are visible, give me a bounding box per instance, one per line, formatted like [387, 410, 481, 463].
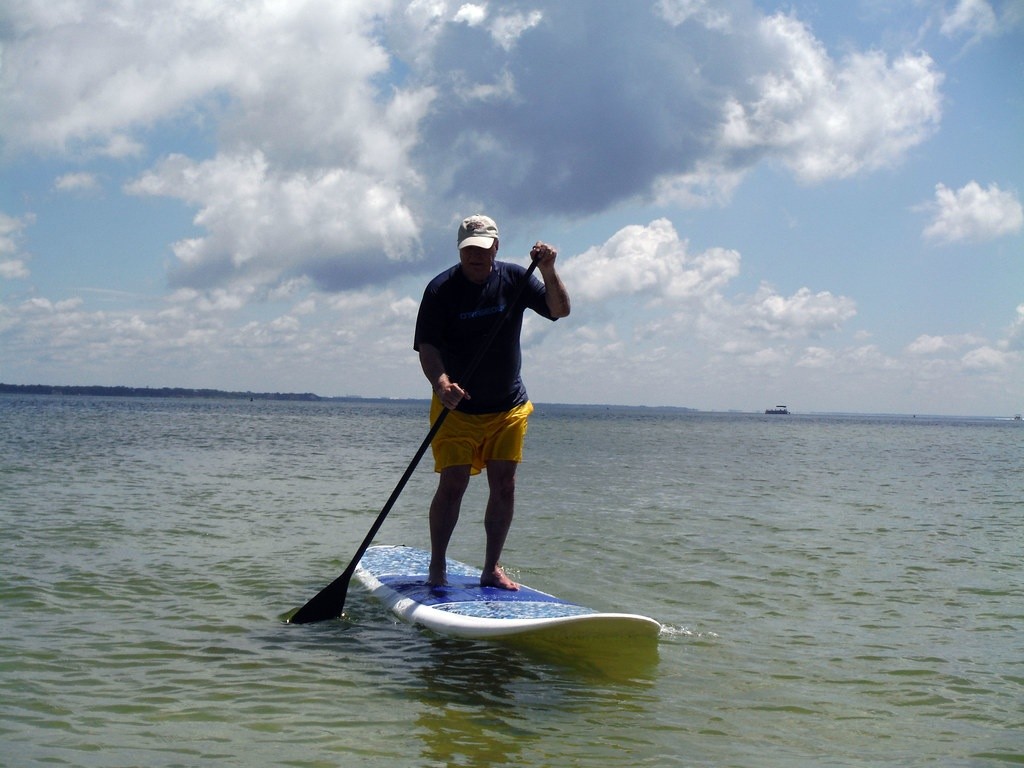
[456, 213, 499, 250]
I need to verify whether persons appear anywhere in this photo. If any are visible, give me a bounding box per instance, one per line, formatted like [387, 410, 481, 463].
[413, 214, 571, 591]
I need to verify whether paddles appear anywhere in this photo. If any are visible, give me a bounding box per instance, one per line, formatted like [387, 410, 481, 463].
[284, 245, 549, 626]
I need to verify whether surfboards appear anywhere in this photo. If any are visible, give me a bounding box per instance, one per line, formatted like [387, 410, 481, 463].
[353, 543, 664, 641]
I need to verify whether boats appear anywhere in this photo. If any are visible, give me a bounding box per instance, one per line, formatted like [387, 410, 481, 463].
[765, 405, 790, 414]
